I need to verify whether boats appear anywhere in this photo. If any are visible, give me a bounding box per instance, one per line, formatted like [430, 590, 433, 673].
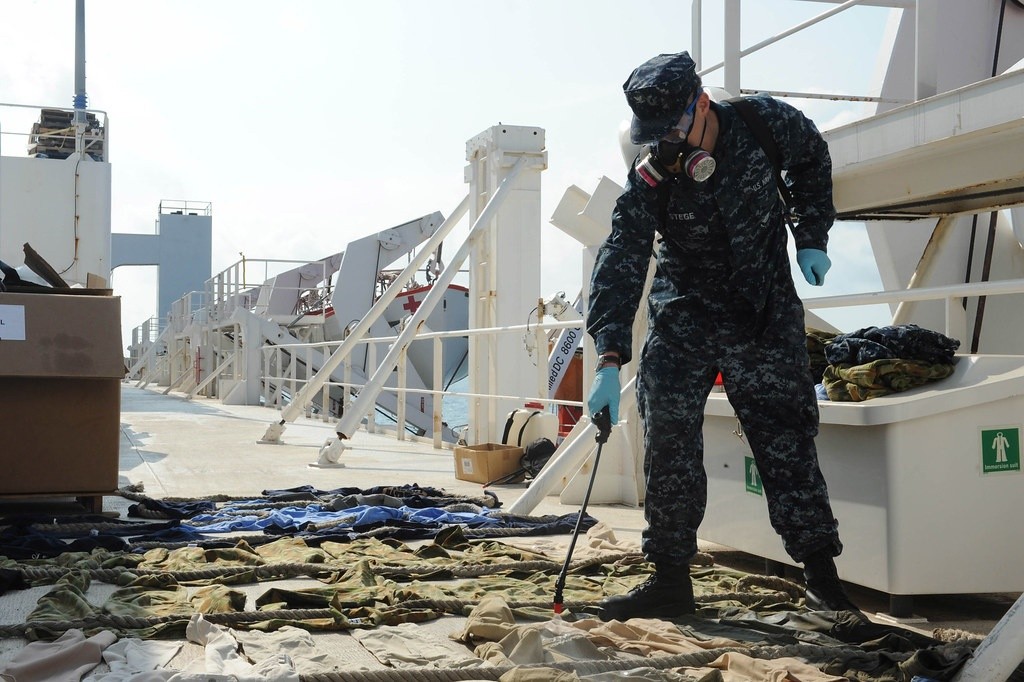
[305, 283, 469, 390]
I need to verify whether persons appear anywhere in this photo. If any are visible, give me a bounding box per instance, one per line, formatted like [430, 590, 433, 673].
[585, 51, 861, 623]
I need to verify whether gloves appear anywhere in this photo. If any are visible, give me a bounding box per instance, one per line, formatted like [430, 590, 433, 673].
[588, 366, 620, 425]
[796, 249, 831, 286]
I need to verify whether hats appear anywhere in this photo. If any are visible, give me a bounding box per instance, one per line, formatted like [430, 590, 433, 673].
[623, 51, 701, 145]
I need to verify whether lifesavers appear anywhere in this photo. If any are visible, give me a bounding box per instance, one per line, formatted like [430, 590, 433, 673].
[431, 259, 444, 275]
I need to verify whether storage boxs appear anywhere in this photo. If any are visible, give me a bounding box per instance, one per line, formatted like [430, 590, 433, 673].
[454, 442, 526, 485]
[0, 292, 125, 494]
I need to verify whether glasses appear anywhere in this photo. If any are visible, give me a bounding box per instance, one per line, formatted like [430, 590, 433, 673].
[642, 111, 692, 147]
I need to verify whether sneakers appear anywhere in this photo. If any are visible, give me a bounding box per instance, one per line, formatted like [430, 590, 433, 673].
[598, 563, 695, 622]
[805, 559, 872, 633]
